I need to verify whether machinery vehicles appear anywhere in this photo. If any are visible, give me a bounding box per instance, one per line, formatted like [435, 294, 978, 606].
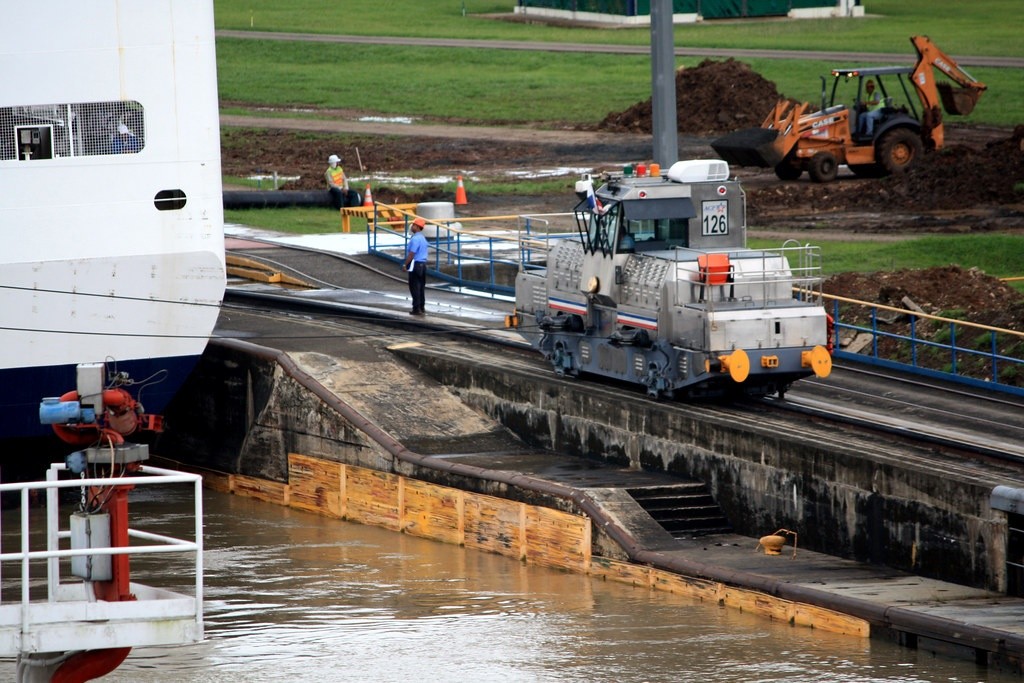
[510, 157, 831, 401]
[710, 33, 988, 181]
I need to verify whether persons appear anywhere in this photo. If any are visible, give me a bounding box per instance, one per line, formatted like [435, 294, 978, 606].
[401, 218, 428, 315]
[856, 81, 884, 136]
[324, 154, 359, 207]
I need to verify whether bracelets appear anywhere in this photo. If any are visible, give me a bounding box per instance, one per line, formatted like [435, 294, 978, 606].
[403, 264, 406, 267]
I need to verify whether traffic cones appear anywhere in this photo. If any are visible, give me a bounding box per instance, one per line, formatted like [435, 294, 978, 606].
[454, 175, 469, 205]
[363, 184, 374, 207]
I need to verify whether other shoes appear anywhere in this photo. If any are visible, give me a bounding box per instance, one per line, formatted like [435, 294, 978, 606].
[409, 309, 424, 315]
[860, 134, 872, 140]
[850, 131, 861, 138]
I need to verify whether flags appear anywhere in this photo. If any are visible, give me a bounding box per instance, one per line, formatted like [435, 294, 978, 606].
[575, 180, 609, 215]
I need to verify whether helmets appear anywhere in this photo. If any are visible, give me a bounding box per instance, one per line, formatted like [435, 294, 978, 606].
[866, 80, 874, 86]
[327, 155, 340, 163]
[413, 218, 426, 228]
